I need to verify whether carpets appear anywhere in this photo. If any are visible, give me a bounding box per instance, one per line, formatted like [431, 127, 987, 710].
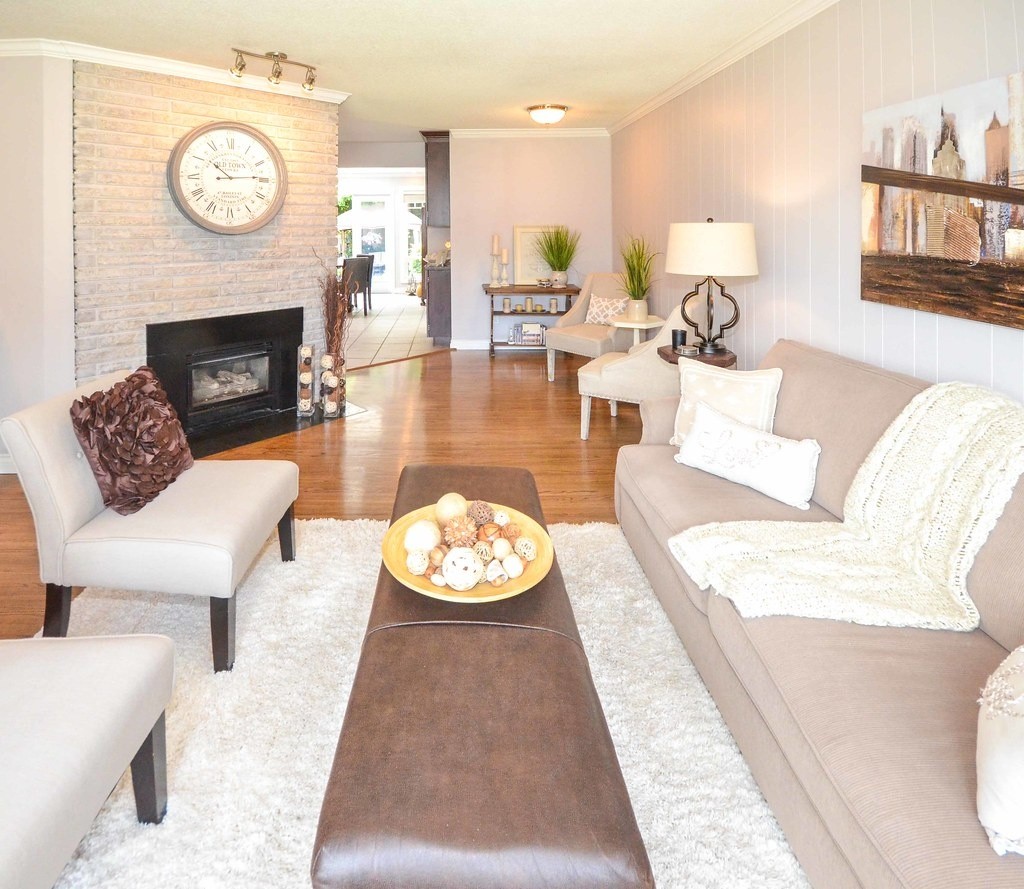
[33, 517, 815, 889]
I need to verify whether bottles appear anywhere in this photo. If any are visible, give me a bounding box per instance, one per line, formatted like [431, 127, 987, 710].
[417, 281, 423, 297]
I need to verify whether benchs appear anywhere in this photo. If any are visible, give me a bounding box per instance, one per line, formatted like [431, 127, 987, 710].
[0, 633, 176, 889]
[0, 365, 301, 675]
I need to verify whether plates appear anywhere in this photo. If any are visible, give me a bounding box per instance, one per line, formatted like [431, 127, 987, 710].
[382, 500, 553, 603]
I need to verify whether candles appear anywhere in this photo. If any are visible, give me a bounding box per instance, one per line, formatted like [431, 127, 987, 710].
[501, 248, 509, 263]
[491, 234, 500, 255]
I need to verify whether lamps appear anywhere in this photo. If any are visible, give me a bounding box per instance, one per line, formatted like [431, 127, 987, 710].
[227, 51, 246, 82]
[527, 104, 568, 124]
[299, 67, 318, 98]
[665, 218, 759, 354]
[265, 60, 284, 91]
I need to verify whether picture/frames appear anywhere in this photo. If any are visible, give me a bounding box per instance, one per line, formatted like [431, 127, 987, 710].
[513, 224, 560, 285]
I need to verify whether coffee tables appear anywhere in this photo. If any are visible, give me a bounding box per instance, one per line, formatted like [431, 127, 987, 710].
[311, 463, 658, 889]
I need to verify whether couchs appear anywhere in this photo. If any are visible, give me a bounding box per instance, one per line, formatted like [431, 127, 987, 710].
[612, 338, 1024, 889]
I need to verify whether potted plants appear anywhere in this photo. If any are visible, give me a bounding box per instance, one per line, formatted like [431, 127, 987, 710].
[530, 224, 584, 289]
[609, 227, 664, 320]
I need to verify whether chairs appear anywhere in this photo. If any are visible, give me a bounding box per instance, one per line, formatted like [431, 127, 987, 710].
[337, 255, 374, 310]
[577, 293, 707, 441]
[337, 257, 369, 316]
[546, 271, 647, 382]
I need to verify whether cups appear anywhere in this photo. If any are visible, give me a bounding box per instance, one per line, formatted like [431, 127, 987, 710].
[672, 329, 686, 349]
[536, 304, 543, 312]
[550, 298, 557, 314]
[525, 297, 533, 312]
[515, 304, 523, 312]
[503, 298, 510, 313]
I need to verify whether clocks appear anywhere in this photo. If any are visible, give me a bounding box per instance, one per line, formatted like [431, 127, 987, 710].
[167, 119, 289, 235]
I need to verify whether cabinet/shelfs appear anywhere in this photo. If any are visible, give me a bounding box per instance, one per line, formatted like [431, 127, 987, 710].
[419, 131, 450, 227]
[424, 263, 451, 347]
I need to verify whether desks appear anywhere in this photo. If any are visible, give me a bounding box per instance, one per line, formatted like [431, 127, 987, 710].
[482, 284, 581, 357]
[606, 315, 665, 346]
[657, 346, 738, 369]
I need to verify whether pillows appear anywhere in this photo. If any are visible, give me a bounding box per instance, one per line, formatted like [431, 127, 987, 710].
[583, 293, 630, 325]
[674, 398, 822, 510]
[69, 366, 193, 516]
[669, 356, 784, 448]
